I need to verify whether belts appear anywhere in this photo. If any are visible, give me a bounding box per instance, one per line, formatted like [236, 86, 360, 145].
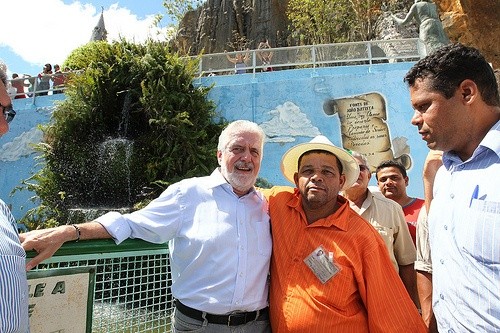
[175, 299, 269, 328]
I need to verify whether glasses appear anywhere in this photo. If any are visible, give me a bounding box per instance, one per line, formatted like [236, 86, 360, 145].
[0, 103, 16, 123]
[360, 164, 369, 171]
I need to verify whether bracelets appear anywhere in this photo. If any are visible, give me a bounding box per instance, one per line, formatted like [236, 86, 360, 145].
[71, 224, 81, 243]
[417, 308, 422, 314]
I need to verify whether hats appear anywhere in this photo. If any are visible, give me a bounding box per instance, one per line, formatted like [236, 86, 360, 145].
[280, 135, 360, 191]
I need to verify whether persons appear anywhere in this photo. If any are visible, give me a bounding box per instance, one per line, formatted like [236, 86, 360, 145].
[254, 135, 429, 333]
[18, 120, 274, 333]
[11, 64, 71, 99]
[339, 147, 445, 331]
[0, 63, 29, 333]
[224, 48, 250, 74]
[384, 0, 450, 60]
[402, 39, 500, 333]
